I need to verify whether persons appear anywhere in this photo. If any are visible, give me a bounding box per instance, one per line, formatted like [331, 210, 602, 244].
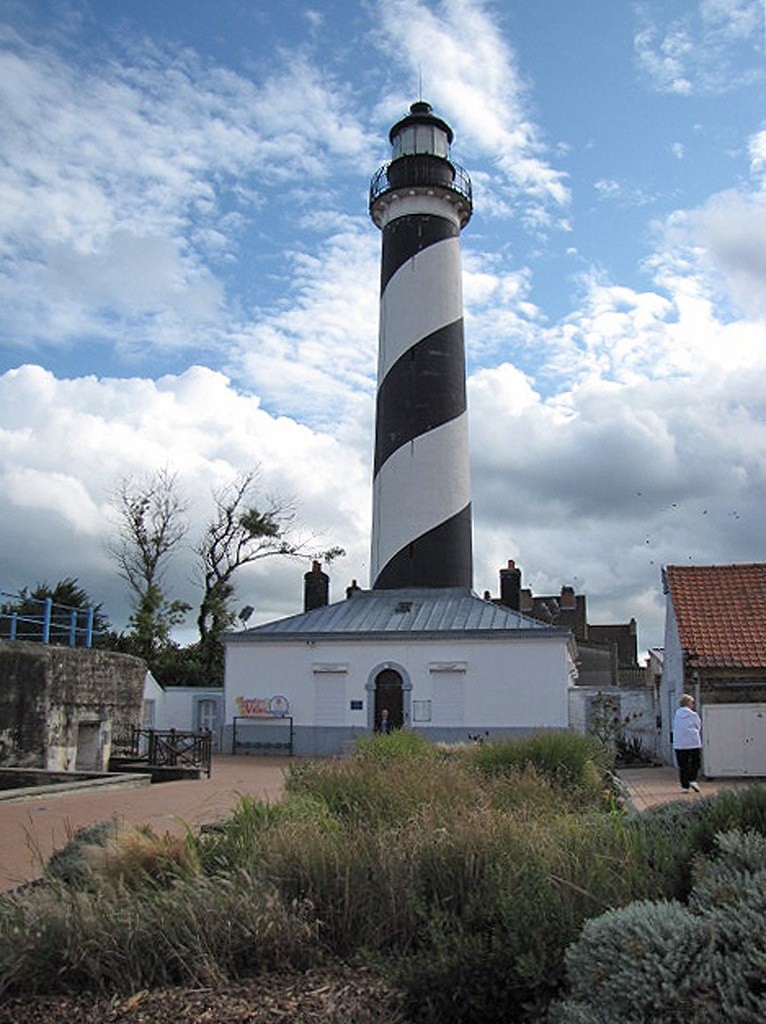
[377, 709, 394, 735]
[674, 694, 703, 793]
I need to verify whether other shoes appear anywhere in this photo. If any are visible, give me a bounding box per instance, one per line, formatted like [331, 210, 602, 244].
[683, 787, 689, 793]
[691, 781, 701, 792]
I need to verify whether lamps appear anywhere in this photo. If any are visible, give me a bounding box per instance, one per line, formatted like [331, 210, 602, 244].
[548, 605, 576, 625]
[238, 606, 254, 633]
